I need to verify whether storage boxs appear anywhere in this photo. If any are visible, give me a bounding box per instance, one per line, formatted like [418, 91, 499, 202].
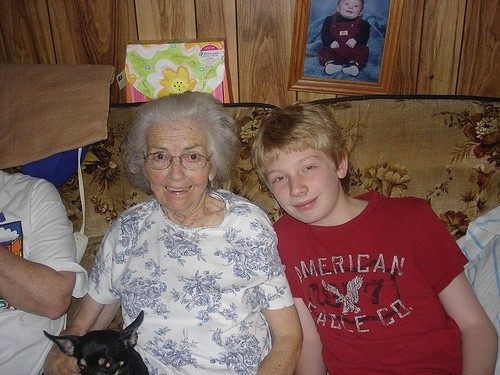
[126, 41, 231, 105]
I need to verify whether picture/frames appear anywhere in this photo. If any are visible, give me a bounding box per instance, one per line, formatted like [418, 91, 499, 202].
[287, 0, 403, 95]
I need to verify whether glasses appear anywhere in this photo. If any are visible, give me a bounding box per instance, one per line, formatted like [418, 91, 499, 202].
[145, 151, 214, 171]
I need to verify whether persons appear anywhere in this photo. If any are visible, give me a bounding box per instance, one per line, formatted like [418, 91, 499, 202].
[0, 168, 89, 375]
[252, 102, 500, 375]
[318, 0, 371, 76]
[43, 92, 304, 375]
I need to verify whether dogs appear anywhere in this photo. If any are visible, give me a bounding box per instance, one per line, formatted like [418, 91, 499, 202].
[40, 311, 152, 375]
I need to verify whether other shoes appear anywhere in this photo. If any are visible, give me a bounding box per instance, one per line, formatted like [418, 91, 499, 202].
[325, 64, 343, 75]
[342, 64, 359, 77]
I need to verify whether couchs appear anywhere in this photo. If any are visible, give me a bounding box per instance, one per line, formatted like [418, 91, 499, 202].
[5, 96, 500, 343]
